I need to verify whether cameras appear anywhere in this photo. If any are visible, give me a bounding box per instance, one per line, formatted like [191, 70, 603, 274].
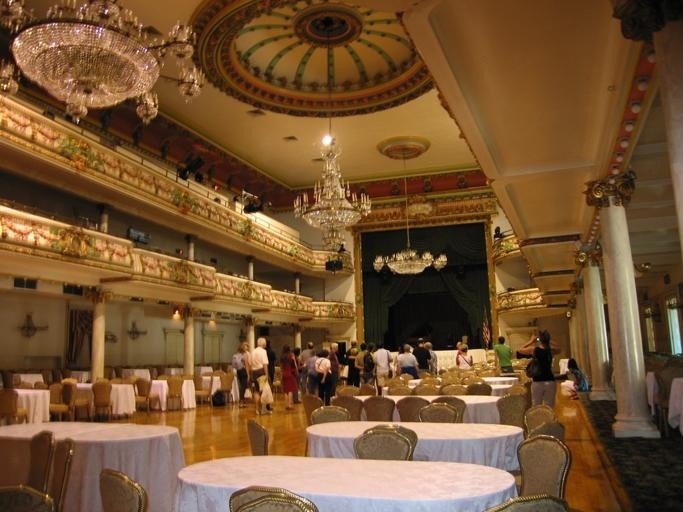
[537, 337, 540, 342]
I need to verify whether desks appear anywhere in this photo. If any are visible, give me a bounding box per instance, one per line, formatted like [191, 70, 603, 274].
[176, 455, 518, 511]
[1, 421, 186, 512]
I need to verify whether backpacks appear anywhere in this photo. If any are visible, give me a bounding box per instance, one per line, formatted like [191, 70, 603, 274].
[231, 352, 244, 370]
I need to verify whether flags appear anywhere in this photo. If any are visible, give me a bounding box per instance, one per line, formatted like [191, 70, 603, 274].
[482, 312, 492, 349]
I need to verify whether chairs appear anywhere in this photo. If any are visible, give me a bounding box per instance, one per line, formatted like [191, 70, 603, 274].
[47, 437, 74, 511]
[26, 431, 54, 491]
[611, 349, 682, 437]
[1, 359, 235, 424]
[230, 486, 318, 512]
[515, 435, 572, 501]
[247, 352, 566, 473]
[488, 493, 569, 512]
[100, 469, 147, 512]
[1, 484, 55, 511]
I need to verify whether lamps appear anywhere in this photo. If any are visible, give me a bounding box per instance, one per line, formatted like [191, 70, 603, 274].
[292, 26, 373, 256]
[370, 148, 450, 277]
[0, 1, 207, 127]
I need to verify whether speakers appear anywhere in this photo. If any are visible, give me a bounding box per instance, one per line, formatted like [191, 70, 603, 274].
[326, 261, 343, 270]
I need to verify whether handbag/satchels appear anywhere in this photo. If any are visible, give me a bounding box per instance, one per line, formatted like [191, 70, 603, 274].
[526, 357, 541, 378]
[363, 352, 374, 373]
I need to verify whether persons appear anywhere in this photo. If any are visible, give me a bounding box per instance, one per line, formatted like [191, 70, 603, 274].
[567, 358, 589, 400]
[456, 341, 473, 369]
[518, 329, 557, 409]
[236, 337, 437, 414]
[493, 336, 514, 373]
